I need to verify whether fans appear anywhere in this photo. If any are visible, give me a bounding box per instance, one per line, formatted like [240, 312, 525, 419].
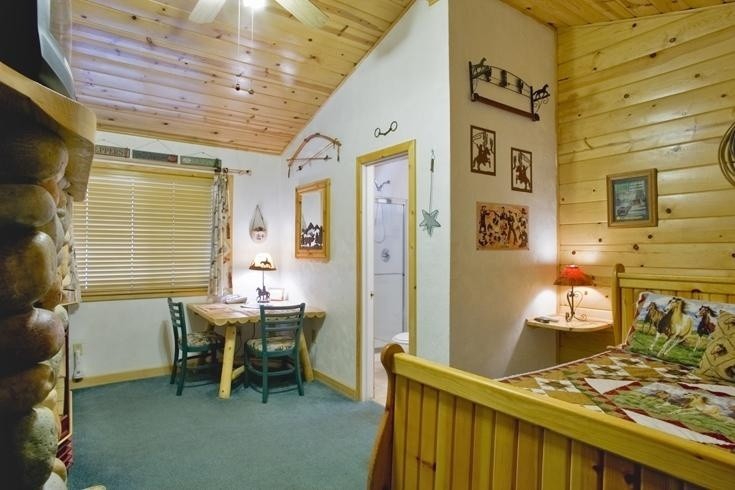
[186, 0, 332, 31]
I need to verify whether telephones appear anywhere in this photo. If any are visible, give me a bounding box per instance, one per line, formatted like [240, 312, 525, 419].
[224, 295, 247, 304]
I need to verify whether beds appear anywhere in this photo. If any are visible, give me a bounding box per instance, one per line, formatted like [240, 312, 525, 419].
[365, 273, 734, 488]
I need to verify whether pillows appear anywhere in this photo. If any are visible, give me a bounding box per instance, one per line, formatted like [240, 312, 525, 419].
[622, 286, 734, 368]
[700, 310, 734, 385]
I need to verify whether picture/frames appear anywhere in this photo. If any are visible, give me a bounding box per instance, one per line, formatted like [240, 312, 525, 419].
[295, 177, 330, 261]
[604, 167, 659, 230]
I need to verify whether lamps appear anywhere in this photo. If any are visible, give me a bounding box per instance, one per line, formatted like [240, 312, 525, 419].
[554, 264, 594, 322]
[250, 251, 276, 288]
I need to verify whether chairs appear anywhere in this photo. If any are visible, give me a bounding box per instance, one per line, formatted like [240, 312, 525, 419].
[163, 294, 227, 395]
[244, 300, 307, 403]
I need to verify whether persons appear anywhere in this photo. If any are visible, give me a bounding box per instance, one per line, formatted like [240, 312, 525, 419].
[478, 204, 528, 249]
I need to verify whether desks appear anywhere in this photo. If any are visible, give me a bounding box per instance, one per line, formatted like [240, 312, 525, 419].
[187, 297, 326, 400]
